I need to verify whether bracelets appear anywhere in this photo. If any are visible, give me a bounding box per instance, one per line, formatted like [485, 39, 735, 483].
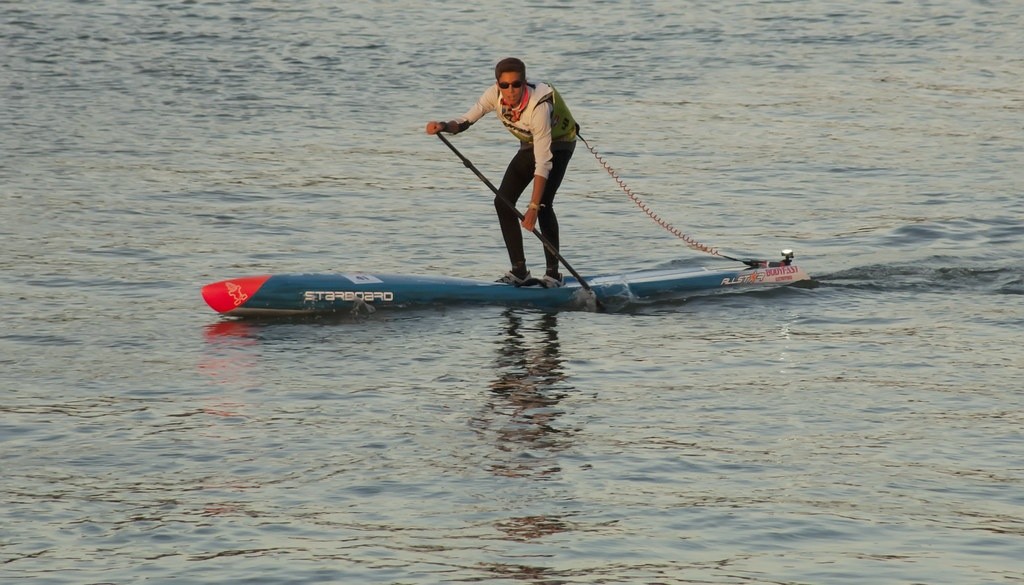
[528, 204, 539, 210]
[440, 121, 449, 131]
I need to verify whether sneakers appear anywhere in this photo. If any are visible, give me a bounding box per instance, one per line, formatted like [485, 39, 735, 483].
[538, 273, 566, 288]
[494, 270, 532, 286]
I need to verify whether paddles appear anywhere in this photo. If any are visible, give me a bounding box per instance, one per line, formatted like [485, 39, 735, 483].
[437, 131, 593, 292]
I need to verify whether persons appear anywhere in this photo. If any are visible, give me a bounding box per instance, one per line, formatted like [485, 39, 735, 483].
[426, 57, 577, 288]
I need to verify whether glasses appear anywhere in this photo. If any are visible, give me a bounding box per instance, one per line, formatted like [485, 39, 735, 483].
[498, 79, 522, 89]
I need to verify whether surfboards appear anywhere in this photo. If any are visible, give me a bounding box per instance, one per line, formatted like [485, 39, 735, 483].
[202, 257, 791, 315]
